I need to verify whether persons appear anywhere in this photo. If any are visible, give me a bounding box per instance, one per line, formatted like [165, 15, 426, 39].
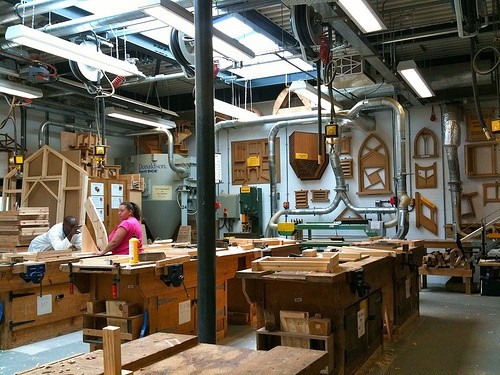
[93, 202, 144, 255]
[28, 215, 83, 251]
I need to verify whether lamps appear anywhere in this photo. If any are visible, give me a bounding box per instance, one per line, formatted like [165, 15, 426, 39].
[0, 79, 43, 99]
[335, 0, 387, 34]
[5, 0, 139, 77]
[289, 74, 343, 112]
[397, 9, 435, 98]
[105, 107, 176, 129]
[139, 0, 255, 63]
